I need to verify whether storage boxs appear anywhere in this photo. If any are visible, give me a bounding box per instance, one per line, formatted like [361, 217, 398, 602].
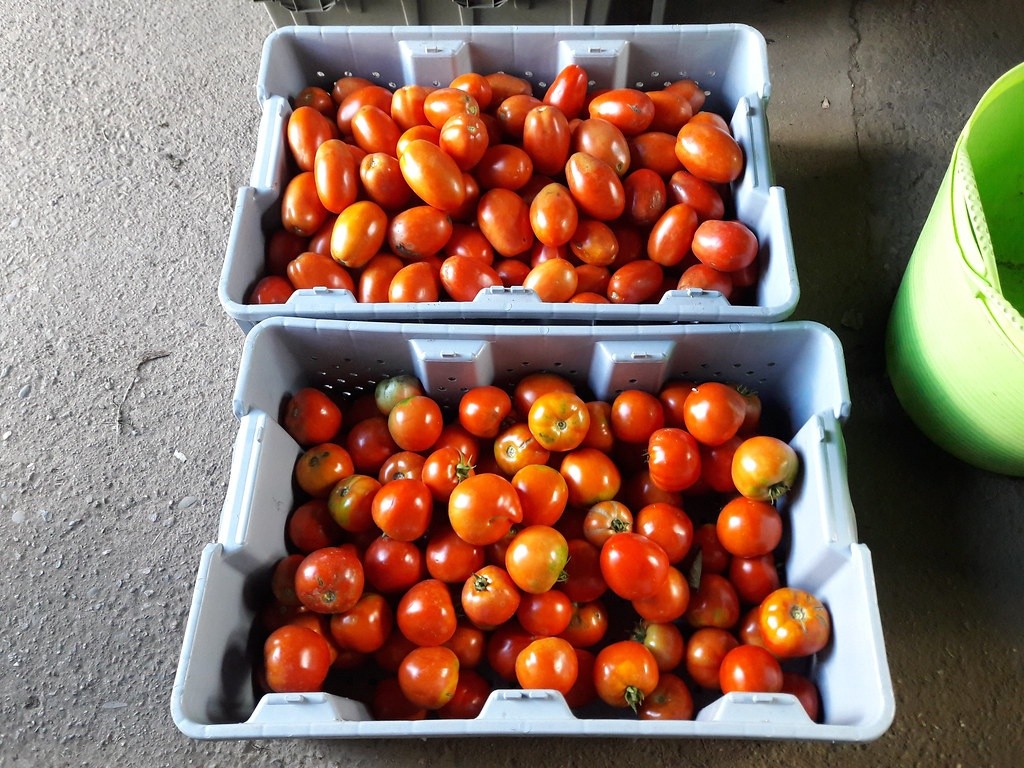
[167, 314, 897, 742]
[219, 24, 801, 322]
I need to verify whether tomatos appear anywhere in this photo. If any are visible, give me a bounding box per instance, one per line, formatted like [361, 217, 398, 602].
[264, 374, 828, 721]
[254, 64, 759, 304]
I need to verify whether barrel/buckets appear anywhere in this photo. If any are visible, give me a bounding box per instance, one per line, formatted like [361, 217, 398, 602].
[884, 61, 1024, 475]
